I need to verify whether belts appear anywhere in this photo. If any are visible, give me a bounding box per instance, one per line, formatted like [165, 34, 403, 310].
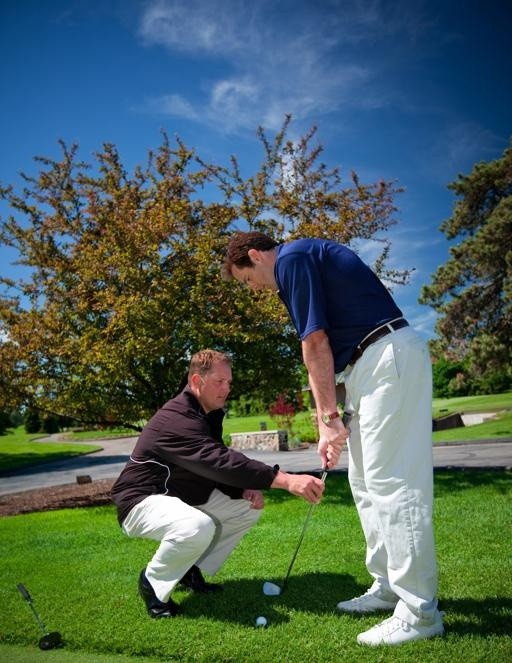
[348, 317, 410, 367]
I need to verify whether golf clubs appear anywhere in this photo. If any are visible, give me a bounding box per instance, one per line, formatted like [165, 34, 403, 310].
[263, 412, 352, 596]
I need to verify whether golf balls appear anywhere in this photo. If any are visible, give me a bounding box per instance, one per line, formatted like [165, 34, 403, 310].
[255, 616, 268, 627]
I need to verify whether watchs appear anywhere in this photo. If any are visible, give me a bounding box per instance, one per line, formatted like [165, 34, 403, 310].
[321, 411, 339, 424]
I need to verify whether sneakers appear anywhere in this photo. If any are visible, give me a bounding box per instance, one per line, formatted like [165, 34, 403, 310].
[356, 608, 449, 649]
[337, 587, 395, 612]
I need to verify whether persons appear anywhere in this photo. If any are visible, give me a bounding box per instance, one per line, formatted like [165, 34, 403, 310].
[224, 229, 452, 645]
[110, 349, 326, 618]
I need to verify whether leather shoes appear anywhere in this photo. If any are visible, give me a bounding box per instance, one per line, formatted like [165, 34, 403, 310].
[138, 568, 175, 617]
[180, 566, 221, 593]
